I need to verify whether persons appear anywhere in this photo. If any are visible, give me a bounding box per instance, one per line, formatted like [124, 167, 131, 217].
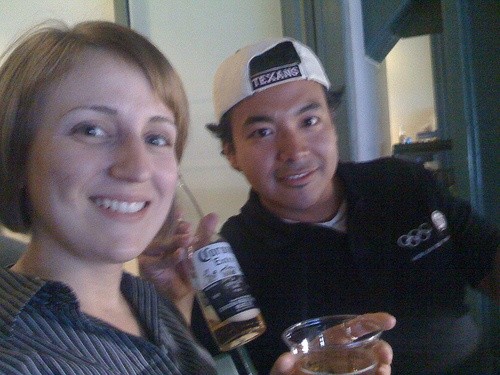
[137, 36, 500, 375]
[0, 18, 397, 375]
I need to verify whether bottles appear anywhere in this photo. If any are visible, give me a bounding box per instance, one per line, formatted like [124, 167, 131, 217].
[175, 176, 266, 351]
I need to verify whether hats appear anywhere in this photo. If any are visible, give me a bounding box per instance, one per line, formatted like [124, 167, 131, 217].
[212, 37, 331, 127]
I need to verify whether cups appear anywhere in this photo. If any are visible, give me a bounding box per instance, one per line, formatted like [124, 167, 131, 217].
[281, 314, 385, 375]
